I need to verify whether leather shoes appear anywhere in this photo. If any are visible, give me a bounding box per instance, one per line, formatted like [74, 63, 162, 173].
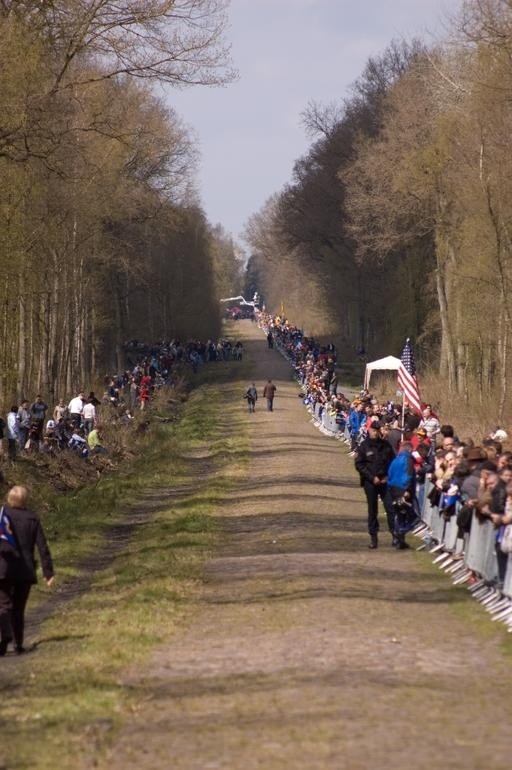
[0, 640, 25, 657]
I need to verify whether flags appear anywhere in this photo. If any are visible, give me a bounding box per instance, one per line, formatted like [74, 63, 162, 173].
[395, 340, 421, 414]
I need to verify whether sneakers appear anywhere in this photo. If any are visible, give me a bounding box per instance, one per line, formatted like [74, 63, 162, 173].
[389, 528, 410, 551]
[483, 578, 504, 590]
[451, 552, 464, 560]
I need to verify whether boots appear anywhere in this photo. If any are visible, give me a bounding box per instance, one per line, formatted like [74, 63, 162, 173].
[369, 533, 377, 548]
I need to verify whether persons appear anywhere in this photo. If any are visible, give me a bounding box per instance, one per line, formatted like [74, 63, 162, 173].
[224, 307, 257, 322]
[1, 333, 245, 460]
[244, 383, 259, 413]
[263, 380, 276, 412]
[258, 310, 303, 349]
[2, 485, 55, 654]
[421, 424, 511, 594]
[328, 389, 441, 549]
[291, 337, 338, 405]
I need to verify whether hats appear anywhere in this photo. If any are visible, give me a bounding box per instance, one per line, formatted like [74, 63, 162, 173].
[467, 448, 484, 460]
[416, 428, 427, 435]
[371, 420, 381, 430]
